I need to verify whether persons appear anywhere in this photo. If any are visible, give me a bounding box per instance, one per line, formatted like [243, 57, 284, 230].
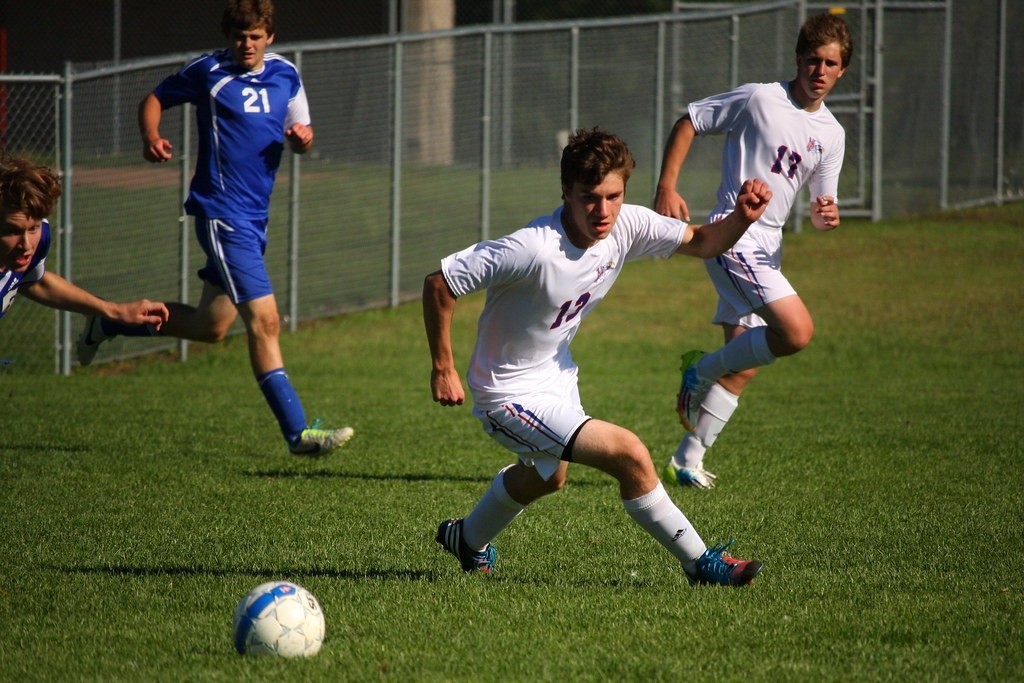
[0, 155, 169, 331]
[423, 126, 772, 589]
[654, 15, 852, 490]
[75, 0, 354, 457]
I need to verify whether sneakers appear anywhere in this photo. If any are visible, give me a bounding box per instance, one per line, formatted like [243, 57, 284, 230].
[288, 424, 353, 454]
[663, 457, 715, 492]
[683, 547, 763, 587]
[76, 300, 108, 366]
[435, 520, 497, 579]
[675, 349, 712, 432]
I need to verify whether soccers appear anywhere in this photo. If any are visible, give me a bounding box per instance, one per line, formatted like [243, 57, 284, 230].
[235, 578, 326, 657]
